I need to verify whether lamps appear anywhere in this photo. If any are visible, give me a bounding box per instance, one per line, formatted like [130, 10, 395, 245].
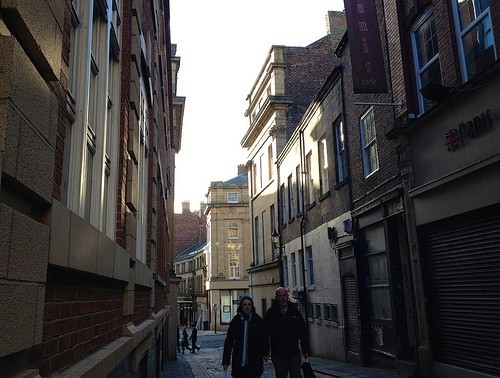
[272, 228, 282, 250]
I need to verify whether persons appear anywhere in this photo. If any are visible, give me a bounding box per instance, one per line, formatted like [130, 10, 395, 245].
[179, 327, 192, 354]
[177, 324, 181, 353]
[188, 324, 200, 353]
[222, 296, 270, 378]
[262, 287, 311, 378]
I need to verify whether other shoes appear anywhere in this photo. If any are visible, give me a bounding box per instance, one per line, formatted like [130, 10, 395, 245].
[198, 348, 200, 352]
[190, 348, 192, 353]
[192, 349, 195, 353]
[177, 351, 181, 353]
[181, 353, 184, 354]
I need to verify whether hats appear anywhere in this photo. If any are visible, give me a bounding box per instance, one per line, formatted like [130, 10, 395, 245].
[239, 295, 253, 305]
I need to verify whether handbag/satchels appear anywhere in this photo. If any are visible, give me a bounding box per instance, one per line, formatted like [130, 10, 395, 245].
[302, 362, 316, 378]
